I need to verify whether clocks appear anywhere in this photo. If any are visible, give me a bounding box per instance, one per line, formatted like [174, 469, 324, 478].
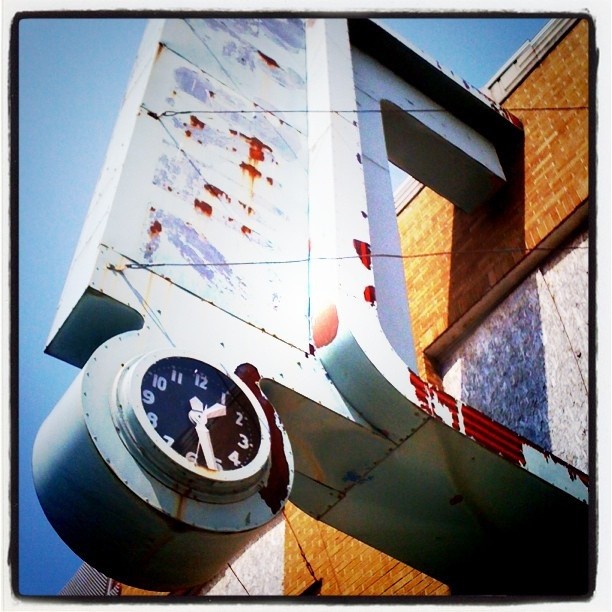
[108, 348, 272, 506]
